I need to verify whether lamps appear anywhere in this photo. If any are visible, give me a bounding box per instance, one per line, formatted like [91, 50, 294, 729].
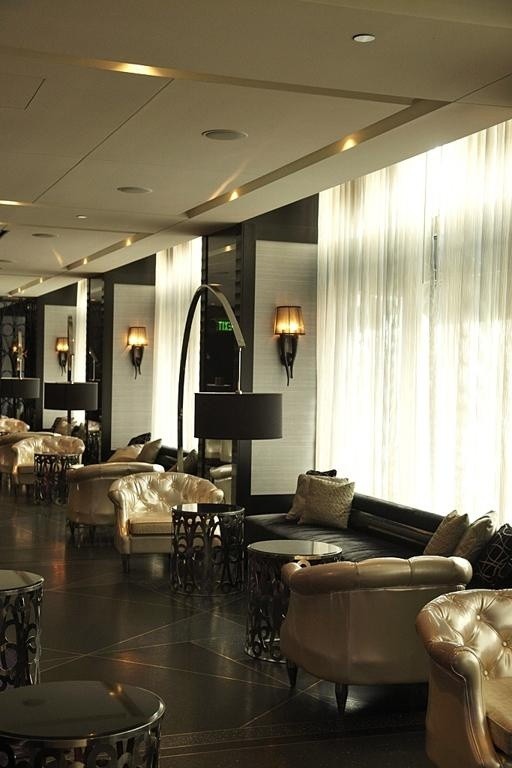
[43, 315, 99, 438]
[177, 283, 284, 474]
[351, 32, 378, 47]
[274, 304, 306, 389]
[126, 325, 149, 380]
[54, 336, 70, 377]
[0, 331, 41, 419]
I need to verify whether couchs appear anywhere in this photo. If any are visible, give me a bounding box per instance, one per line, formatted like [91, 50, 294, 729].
[10, 434, 87, 505]
[414, 587, 511, 767]
[0, 412, 30, 436]
[278, 554, 474, 723]
[107, 471, 226, 574]
[0, 431, 62, 497]
[239, 491, 446, 585]
[64, 462, 166, 553]
[209, 462, 234, 506]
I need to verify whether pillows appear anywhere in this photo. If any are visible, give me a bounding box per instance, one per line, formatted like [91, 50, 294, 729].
[136, 438, 163, 465]
[420, 508, 470, 559]
[465, 522, 511, 589]
[127, 431, 152, 446]
[166, 449, 199, 475]
[450, 508, 497, 566]
[296, 477, 356, 530]
[284, 473, 351, 522]
[306, 468, 339, 476]
[105, 443, 146, 462]
[55, 420, 78, 437]
[51, 417, 61, 433]
